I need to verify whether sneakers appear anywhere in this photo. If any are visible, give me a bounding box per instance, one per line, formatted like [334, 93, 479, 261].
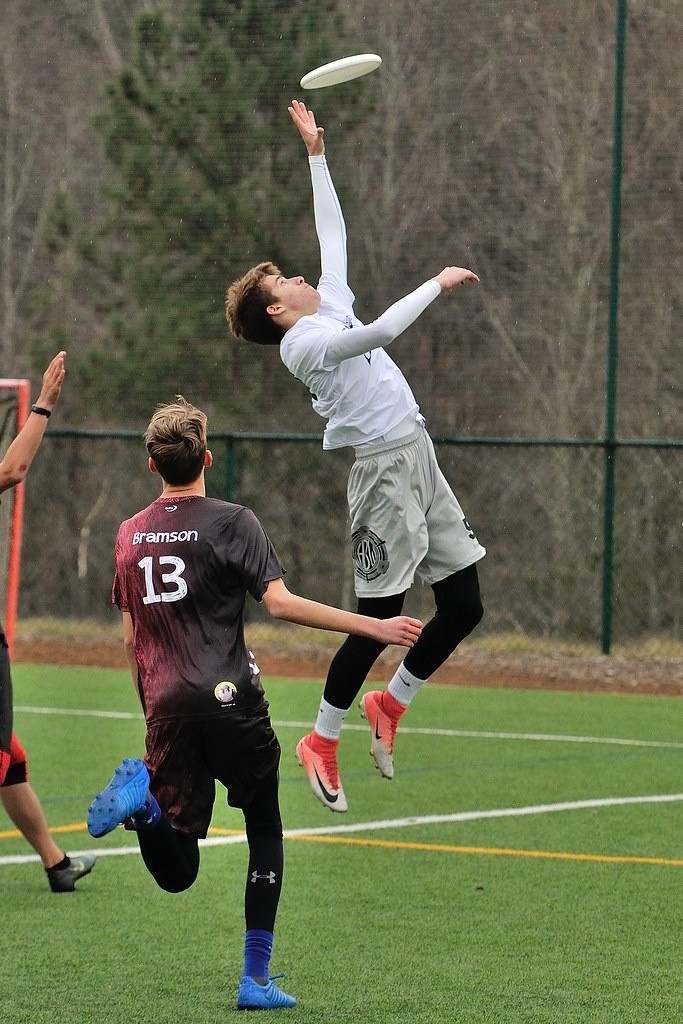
[294, 730, 350, 812]
[360, 690, 408, 780]
[237, 973, 297, 1011]
[45, 856, 96, 893]
[87, 758, 151, 838]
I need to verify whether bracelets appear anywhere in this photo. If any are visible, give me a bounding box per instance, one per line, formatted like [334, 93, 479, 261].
[31, 405, 51, 418]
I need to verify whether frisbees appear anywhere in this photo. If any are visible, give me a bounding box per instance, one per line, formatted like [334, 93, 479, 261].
[300, 54, 382, 90]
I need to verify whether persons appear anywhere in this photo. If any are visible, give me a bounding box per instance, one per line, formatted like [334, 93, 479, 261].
[1, 351, 96, 892]
[227, 100, 487, 813]
[88, 395, 422, 1008]
